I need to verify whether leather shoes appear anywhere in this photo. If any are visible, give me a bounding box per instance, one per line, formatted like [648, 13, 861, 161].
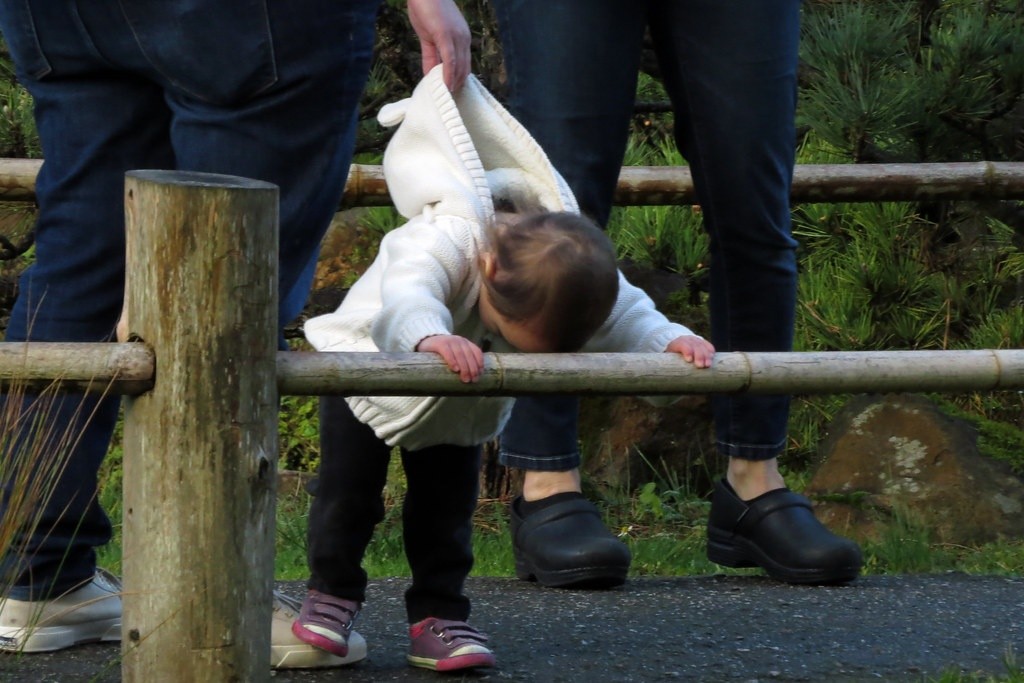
[706, 477, 863, 586]
[509, 496, 631, 588]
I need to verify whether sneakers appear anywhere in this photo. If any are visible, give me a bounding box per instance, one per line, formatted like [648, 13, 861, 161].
[291, 590, 362, 658]
[272, 583, 366, 668]
[0, 566, 123, 653]
[408, 617, 495, 671]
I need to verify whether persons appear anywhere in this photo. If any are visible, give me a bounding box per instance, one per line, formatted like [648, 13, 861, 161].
[291, 57, 716, 672]
[480, 0, 864, 586]
[0, 0, 475, 667]
